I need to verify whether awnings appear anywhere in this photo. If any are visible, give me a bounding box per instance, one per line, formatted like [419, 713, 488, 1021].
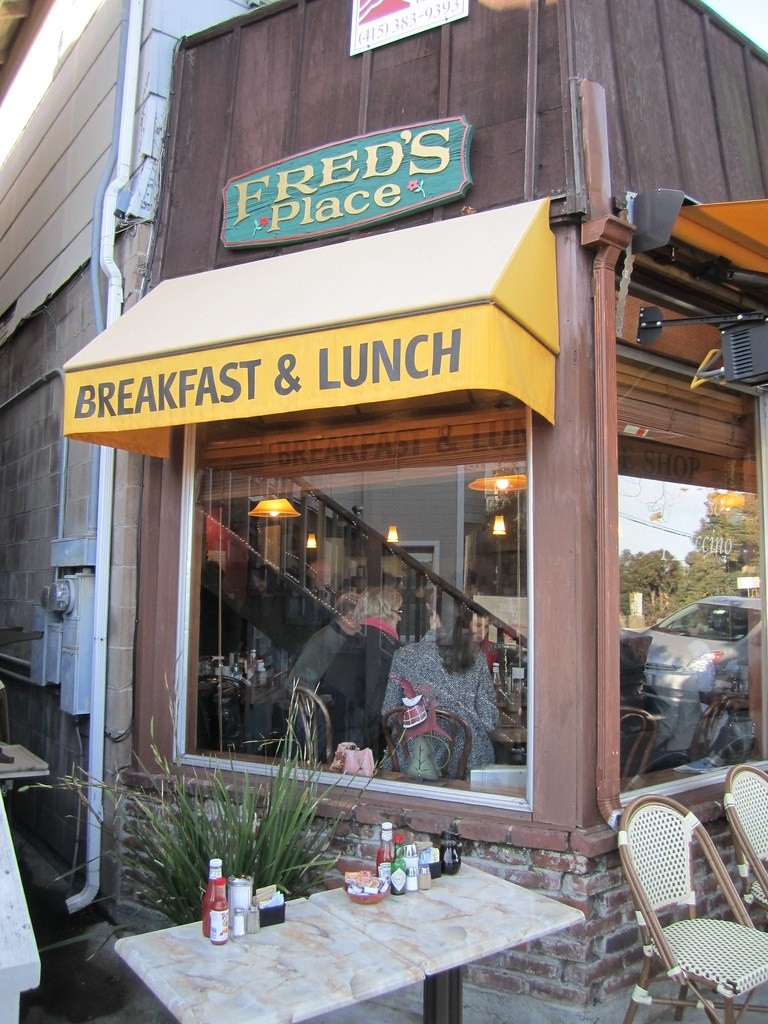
[63, 199, 558, 458]
[648, 199, 768, 326]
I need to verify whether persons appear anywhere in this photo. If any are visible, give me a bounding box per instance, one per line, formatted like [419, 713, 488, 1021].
[286, 577, 499, 778]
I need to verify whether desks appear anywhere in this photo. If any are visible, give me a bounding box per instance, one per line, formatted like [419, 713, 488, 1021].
[113, 863, 586, 1024]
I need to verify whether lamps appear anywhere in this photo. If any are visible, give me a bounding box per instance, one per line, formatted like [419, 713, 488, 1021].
[307, 532, 317, 549]
[493, 515, 506, 535]
[248, 495, 301, 518]
[386, 525, 399, 543]
[468, 442, 528, 492]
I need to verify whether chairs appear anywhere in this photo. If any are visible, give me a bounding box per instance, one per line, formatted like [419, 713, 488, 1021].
[619, 705, 657, 777]
[723, 763, 768, 930]
[296, 686, 332, 765]
[381, 706, 472, 780]
[198, 675, 251, 753]
[690, 693, 749, 763]
[617, 794, 768, 1024]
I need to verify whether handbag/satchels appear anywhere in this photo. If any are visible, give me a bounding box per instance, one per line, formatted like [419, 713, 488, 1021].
[343, 748, 374, 777]
[329, 741, 359, 772]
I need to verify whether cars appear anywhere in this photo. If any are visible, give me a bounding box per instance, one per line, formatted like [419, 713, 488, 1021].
[634, 594, 764, 719]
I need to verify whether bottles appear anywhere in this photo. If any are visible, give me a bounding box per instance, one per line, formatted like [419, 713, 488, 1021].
[209, 877, 229, 945]
[390, 835, 407, 895]
[202, 859, 223, 938]
[402, 843, 431, 892]
[200, 650, 265, 687]
[376, 821, 394, 883]
[226, 874, 254, 928]
[246, 904, 260, 933]
[439, 831, 460, 875]
[491, 662, 501, 702]
[233, 908, 246, 937]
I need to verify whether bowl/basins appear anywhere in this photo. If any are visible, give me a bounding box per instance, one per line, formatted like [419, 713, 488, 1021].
[342, 881, 391, 904]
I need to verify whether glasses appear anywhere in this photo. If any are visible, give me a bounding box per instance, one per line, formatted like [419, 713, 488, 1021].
[390, 608, 402, 615]
